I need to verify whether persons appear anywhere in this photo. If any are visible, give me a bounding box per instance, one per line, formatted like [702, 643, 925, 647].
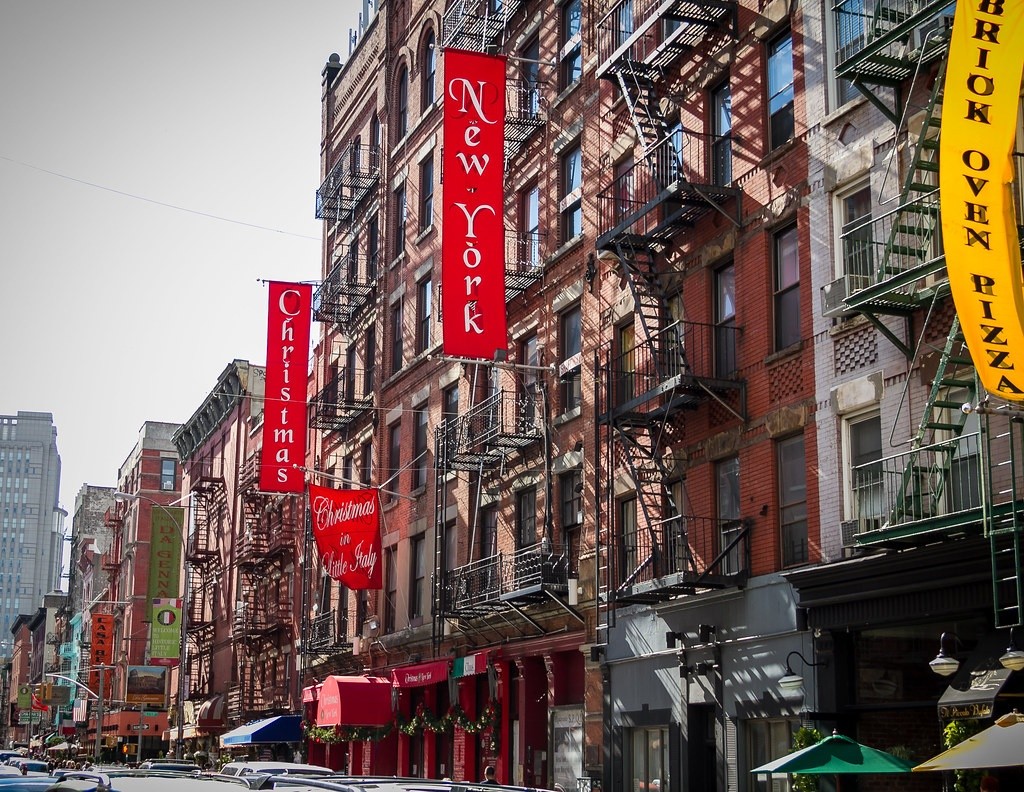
[259, 744, 273, 760]
[21, 751, 92, 771]
[980, 776, 998, 792]
[480, 766, 499, 784]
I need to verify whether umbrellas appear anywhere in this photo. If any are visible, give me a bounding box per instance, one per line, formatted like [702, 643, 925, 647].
[48, 742, 77, 750]
[913, 709, 1024, 772]
[750, 729, 918, 792]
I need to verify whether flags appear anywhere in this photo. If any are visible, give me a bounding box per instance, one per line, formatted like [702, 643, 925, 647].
[307, 482, 383, 589]
[32, 694, 48, 711]
[73, 699, 87, 721]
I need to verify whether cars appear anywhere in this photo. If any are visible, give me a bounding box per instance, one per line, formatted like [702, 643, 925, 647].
[0, 776, 59, 792]
[0, 751, 48, 774]
[0, 765, 23, 777]
[220, 762, 336, 777]
[49, 768, 79, 777]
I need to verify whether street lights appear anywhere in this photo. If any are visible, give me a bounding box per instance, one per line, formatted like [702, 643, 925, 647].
[113, 492, 189, 763]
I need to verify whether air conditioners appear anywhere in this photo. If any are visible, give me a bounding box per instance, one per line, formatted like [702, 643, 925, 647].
[820, 275, 873, 318]
[913, 14, 954, 49]
[838, 514, 886, 549]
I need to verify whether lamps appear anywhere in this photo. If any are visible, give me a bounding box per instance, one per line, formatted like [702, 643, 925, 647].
[929, 632, 965, 676]
[777, 650, 826, 689]
[999, 628, 1024, 671]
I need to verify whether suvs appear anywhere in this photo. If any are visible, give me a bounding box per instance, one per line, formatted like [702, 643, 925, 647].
[43, 770, 361, 792]
[139, 758, 202, 772]
[313, 774, 557, 792]
[86, 764, 128, 771]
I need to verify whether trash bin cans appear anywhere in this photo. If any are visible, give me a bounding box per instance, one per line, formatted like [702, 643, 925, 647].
[126, 753, 137, 762]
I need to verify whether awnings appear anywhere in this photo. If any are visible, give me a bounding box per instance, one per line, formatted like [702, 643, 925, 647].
[938, 669, 1024, 721]
[452, 651, 493, 678]
[196, 696, 227, 732]
[219, 720, 264, 748]
[170, 728, 211, 739]
[30, 733, 57, 748]
[230, 715, 302, 743]
[317, 676, 394, 726]
[391, 661, 449, 688]
